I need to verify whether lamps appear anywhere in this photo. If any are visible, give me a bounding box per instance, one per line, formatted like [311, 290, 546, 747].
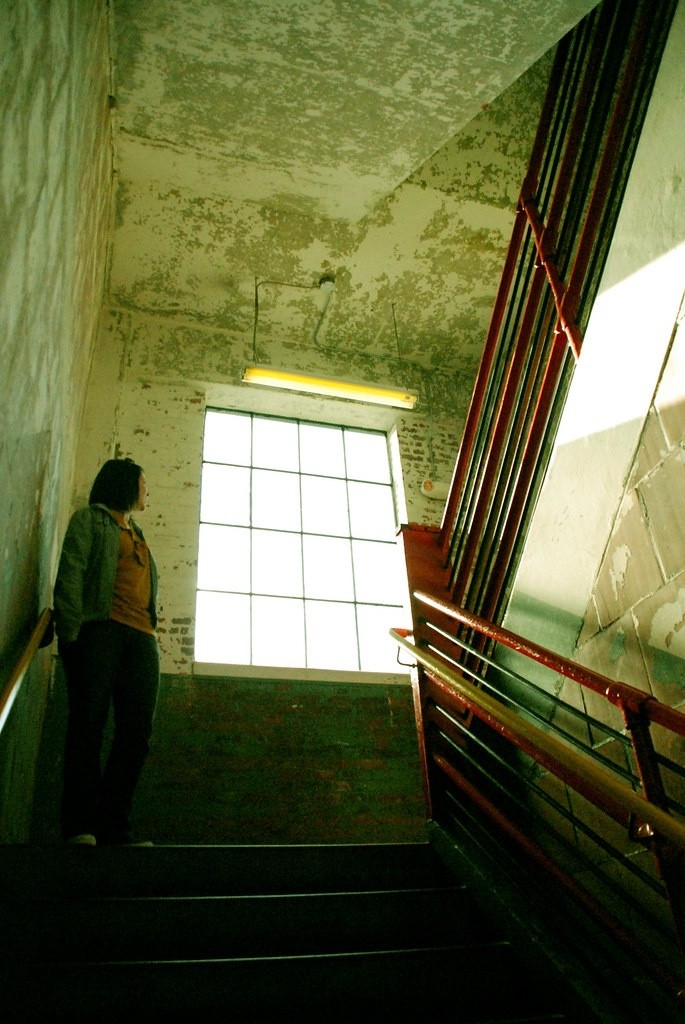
[240, 276, 419, 412]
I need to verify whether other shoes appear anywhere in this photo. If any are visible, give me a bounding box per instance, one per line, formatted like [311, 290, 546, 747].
[65, 833, 96, 847]
[97, 830, 153, 848]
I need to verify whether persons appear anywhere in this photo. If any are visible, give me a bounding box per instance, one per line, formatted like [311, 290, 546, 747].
[54, 457, 159, 847]
